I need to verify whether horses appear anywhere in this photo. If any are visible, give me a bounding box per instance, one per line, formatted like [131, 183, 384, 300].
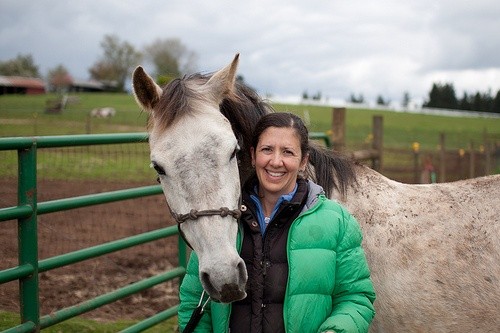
[131, 52, 500, 333]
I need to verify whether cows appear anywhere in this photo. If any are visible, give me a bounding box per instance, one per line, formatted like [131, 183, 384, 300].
[90, 107, 117, 119]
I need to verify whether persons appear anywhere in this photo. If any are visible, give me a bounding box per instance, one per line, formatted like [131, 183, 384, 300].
[176, 113, 377, 333]
